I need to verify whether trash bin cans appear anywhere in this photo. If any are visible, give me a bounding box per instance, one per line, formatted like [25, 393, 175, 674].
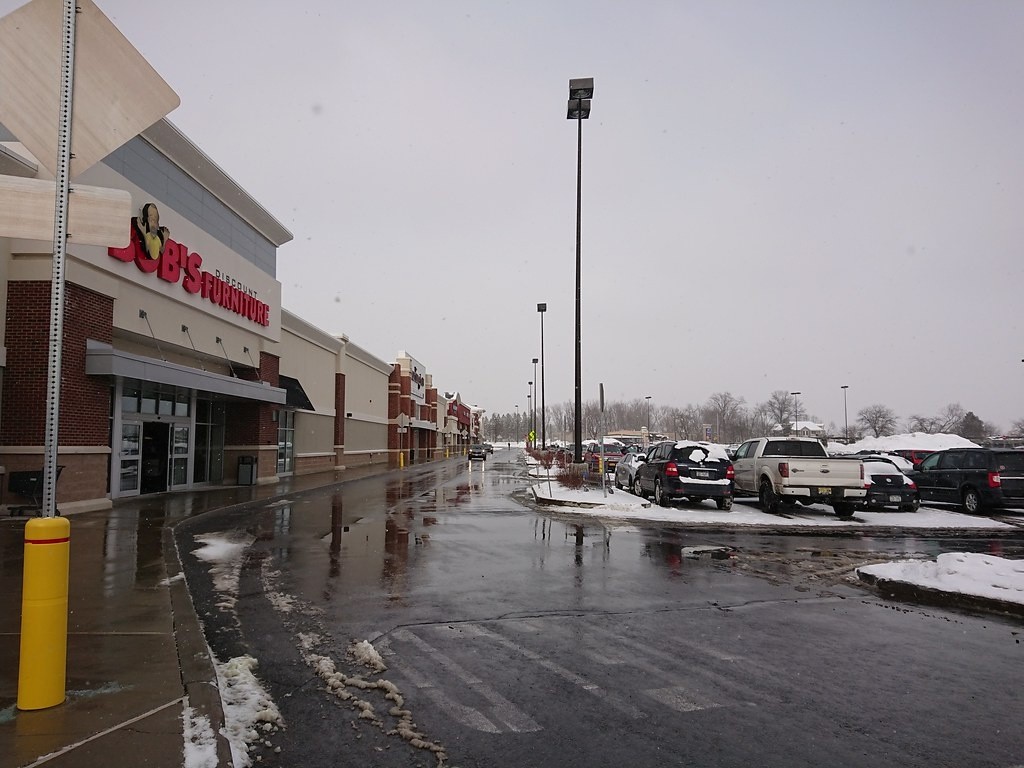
[427, 448, 430, 458]
[410, 449, 415, 460]
[236, 454, 258, 486]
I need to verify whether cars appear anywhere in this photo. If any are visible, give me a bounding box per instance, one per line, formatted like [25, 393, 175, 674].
[467, 444, 487, 461]
[566, 443, 589, 464]
[634, 441, 736, 511]
[843, 454, 920, 513]
[483, 444, 493, 454]
[903, 447, 1024, 514]
[613, 453, 646, 490]
[586, 442, 625, 471]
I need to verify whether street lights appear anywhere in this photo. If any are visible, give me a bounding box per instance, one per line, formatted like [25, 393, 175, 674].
[537, 302, 548, 452]
[526, 395, 531, 449]
[566, 76, 594, 477]
[532, 358, 539, 450]
[528, 381, 534, 449]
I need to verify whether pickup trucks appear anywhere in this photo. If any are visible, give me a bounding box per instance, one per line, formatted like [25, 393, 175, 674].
[731, 436, 865, 518]
